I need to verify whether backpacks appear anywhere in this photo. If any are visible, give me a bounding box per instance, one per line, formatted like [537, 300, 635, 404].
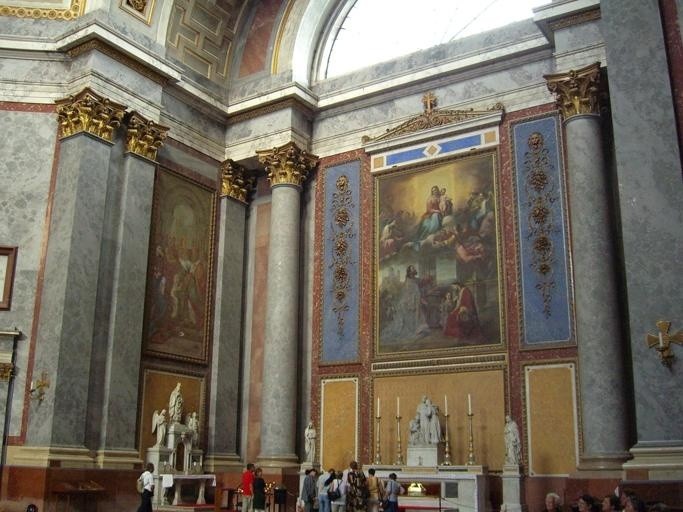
[136, 474, 148, 493]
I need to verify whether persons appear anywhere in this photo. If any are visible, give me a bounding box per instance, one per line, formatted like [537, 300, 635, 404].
[425, 400, 445, 443]
[504, 415, 521, 464]
[253, 468, 266, 512]
[379, 186, 495, 346]
[301, 461, 405, 512]
[416, 396, 430, 444]
[544, 487, 645, 512]
[145, 243, 207, 343]
[169, 383, 183, 423]
[156, 409, 167, 445]
[137, 463, 154, 512]
[304, 422, 317, 462]
[188, 411, 200, 449]
[242, 464, 255, 512]
[408, 413, 419, 445]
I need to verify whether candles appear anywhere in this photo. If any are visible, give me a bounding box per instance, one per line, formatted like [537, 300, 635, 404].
[377, 396, 400, 419]
[444, 393, 472, 416]
[30, 381, 33, 389]
[659, 332, 663, 347]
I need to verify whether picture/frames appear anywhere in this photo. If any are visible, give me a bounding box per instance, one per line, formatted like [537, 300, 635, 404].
[371, 147, 508, 364]
[0, 243, 18, 310]
[135, 161, 220, 471]
[118, 0, 156, 25]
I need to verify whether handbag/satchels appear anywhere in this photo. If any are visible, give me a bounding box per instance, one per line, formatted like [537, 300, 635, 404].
[382, 500, 389, 509]
[327, 478, 341, 500]
[376, 477, 382, 501]
